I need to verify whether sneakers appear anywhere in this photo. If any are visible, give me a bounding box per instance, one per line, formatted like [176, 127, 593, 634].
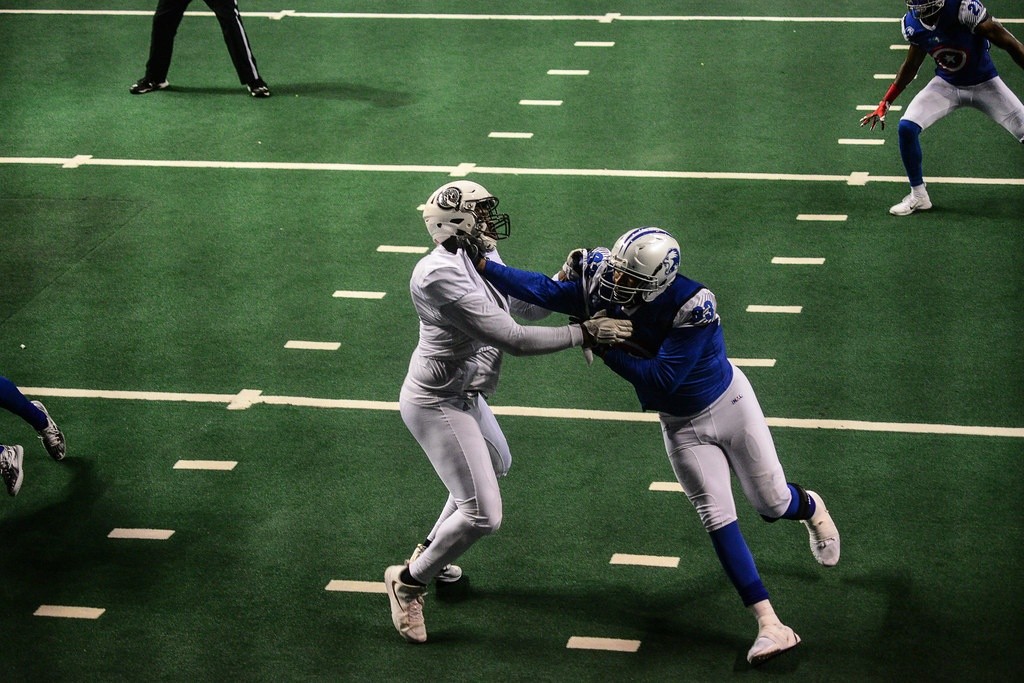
[247, 75, 270, 97]
[385, 565, 427, 643]
[1, 445, 24, 496]
[889, 192, 932, 216]
[800, 490, 840, 567]
[745, 624, 801, 665]
[129, 77, 170, 94]
[410, 543, 462, 583]
[30, 400, 66, 462]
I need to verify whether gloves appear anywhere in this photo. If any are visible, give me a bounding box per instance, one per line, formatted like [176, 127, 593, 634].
[568, 316, 612, 357]
[859, 100, 891, 131]
[457, 234, 484, 269]
[562, 247, 591, 283]
[581, 308, 635, 348]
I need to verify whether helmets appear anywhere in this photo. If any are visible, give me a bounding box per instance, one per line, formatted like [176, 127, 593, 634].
[907, 0, 945, 19]
[598, 226, 681, 305]
[423, 180, 511, 255]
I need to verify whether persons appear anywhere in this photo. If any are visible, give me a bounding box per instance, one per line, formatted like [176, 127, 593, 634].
[858, 0, 1024, 216]
[0, 376, 68, 498]
[128, 0, 271, 97]
[463, 225, 840, 663]
[383, 179, 634, 642]
